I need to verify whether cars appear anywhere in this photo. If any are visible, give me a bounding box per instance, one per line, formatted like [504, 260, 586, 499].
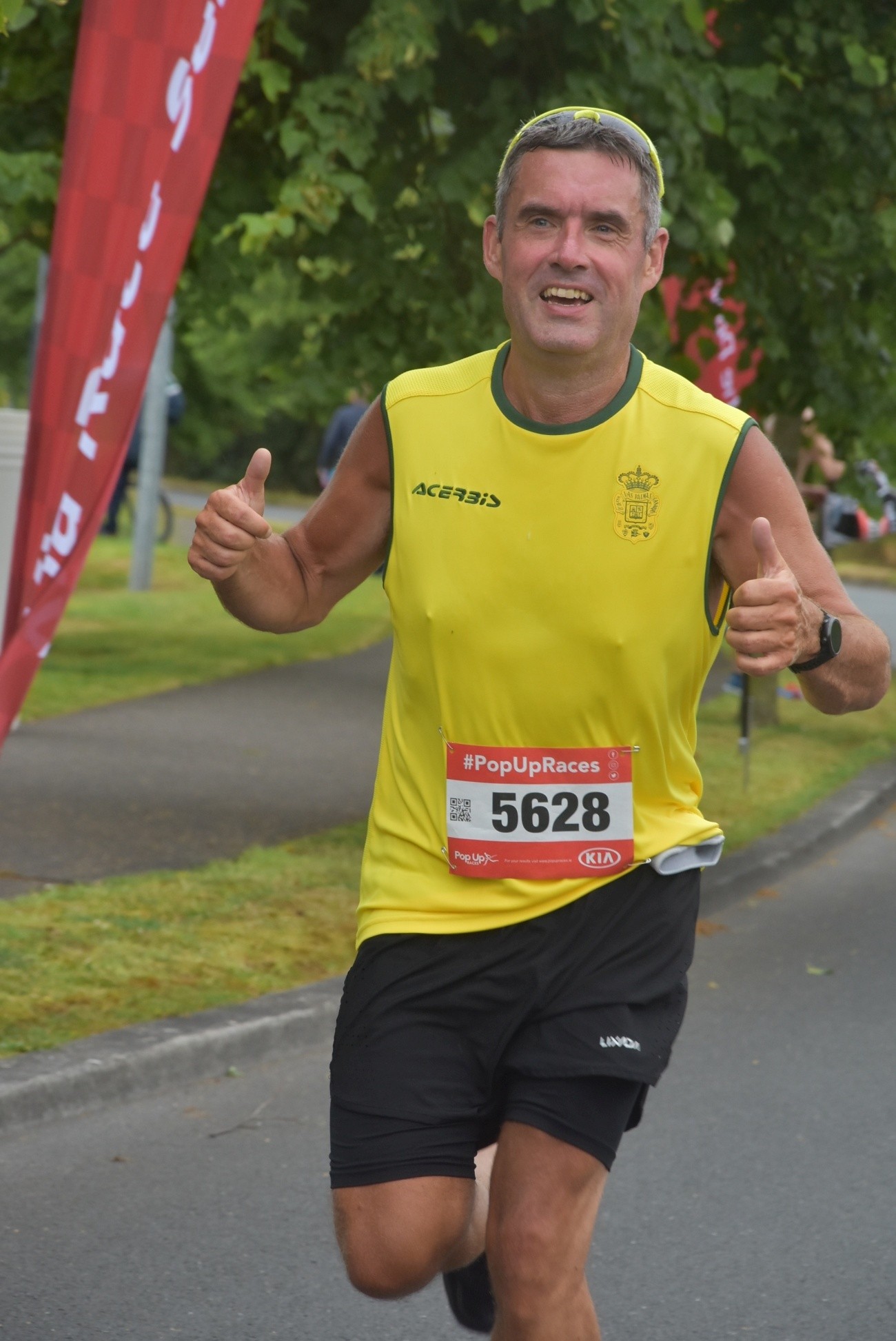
[821, 458, 896, 553]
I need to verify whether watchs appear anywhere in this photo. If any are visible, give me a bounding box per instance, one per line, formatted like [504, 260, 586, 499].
[789, 606, 842, 674]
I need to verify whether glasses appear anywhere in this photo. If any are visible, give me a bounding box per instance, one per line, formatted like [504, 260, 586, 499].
[498, 104, 664, 202]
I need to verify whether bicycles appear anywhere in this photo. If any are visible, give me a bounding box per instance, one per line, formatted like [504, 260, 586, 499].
[114, 482, 173, 543]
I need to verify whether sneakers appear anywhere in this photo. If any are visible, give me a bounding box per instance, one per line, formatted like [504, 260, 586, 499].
[444, 1253, 495, 1331]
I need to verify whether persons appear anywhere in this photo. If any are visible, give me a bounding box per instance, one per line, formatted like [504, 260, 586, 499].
[187, 106, 891, 1341]
[97, 357, 183, 537]
[315, 383, 373, 487]
